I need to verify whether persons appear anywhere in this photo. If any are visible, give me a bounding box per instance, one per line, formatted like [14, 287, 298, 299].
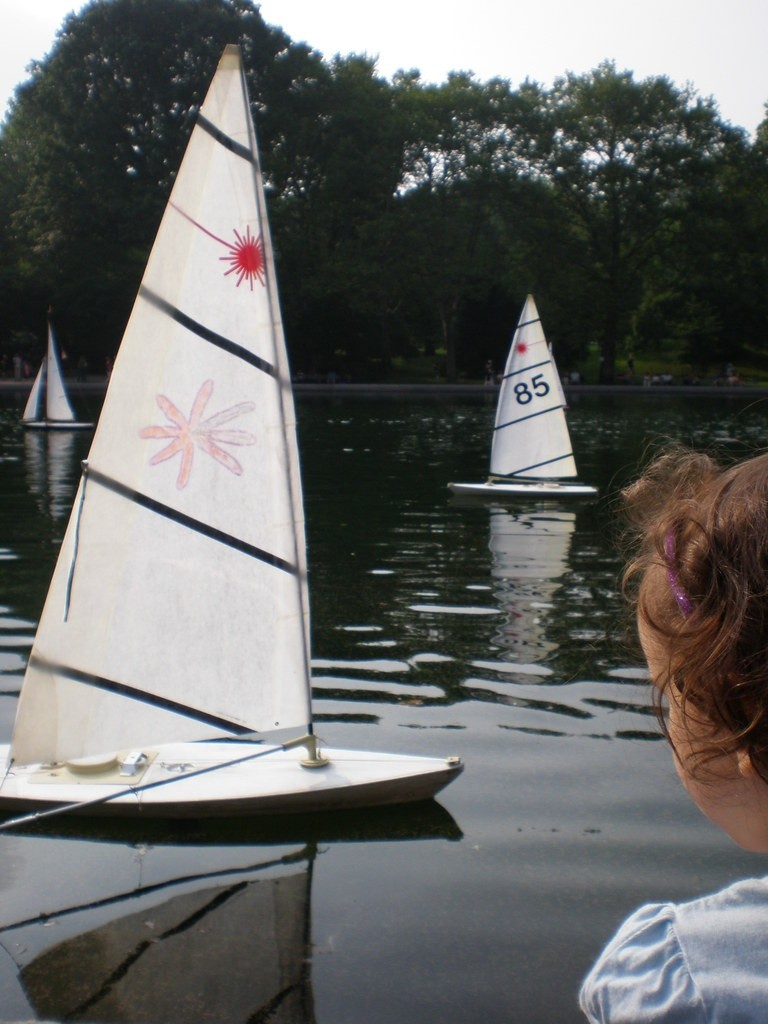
[577, 438, 768, 1024]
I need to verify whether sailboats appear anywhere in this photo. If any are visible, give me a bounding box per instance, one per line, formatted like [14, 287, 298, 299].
[2, 39, 463, 818]
[444, 292, 600, 499]
[1, 801, 464, 1023]
[15, 316, 100, 428]
